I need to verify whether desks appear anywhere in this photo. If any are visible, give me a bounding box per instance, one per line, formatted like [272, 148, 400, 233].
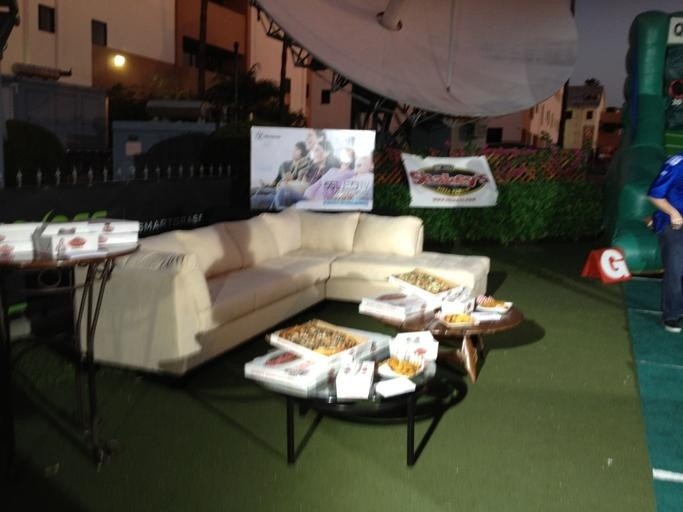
[382, 306, 525, 385]
[0, 243, 143, 472]
[283, 364, 469, 467]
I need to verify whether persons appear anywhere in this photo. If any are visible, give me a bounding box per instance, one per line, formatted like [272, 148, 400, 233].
[251, 128, 374, 210]
[645, 153, 683, 335]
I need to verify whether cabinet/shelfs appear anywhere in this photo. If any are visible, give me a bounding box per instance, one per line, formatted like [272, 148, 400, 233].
[0, 76, 109, 188]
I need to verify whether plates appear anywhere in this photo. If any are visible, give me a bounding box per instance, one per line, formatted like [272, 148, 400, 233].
[476, 301, 513, 313]
[377, 359, 424, 378]
[438, 319, 480, 327]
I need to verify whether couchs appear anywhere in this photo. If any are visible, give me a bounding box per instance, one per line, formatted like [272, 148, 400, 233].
[69, 210, 494, 388]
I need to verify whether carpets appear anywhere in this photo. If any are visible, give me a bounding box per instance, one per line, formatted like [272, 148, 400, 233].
[0, 231, 656, 512]
